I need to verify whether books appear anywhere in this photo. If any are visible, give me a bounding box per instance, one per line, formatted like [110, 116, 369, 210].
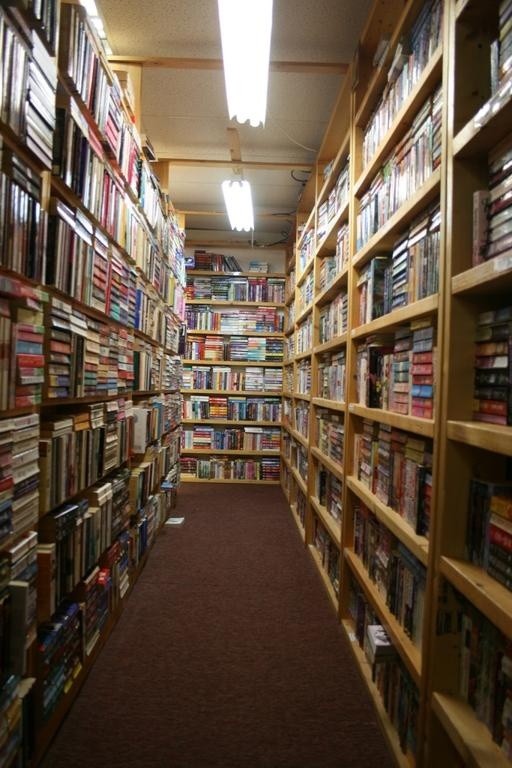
[179, 255, 286, 480]
[1, 0, 186, 767]
[286, 2, 511, 765]
